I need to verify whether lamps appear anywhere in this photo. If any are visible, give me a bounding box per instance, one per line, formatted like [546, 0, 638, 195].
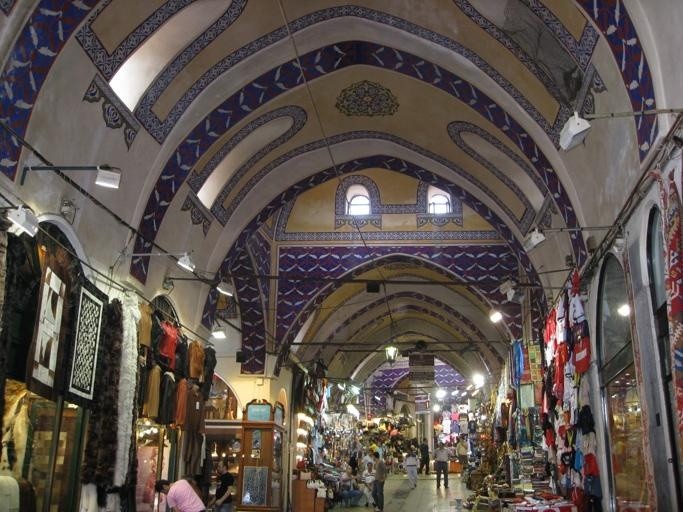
[1, 204, 39, 238]
[559, 108, 683, 151]
[203, 311, 227, 340]
[162, 276, 235, 299]
[522, 226, 611, 254]
[488, 305, 554, 324]
[20, 163, 123, 188]
[499, 279, 572, 296]
[129, 251, 197, 274]
[385, 323, 403, 369]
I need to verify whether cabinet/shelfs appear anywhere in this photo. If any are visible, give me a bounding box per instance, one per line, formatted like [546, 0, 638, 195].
[292, 478, 327, 512]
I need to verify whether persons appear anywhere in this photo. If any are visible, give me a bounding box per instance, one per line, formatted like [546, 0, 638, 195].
[208, 460, 234, 512]
[339, 435, 468, 512]
[155, 479, 207, 512]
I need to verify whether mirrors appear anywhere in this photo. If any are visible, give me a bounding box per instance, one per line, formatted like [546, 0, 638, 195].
[269, 427, 283, 508]
[136, 420, 165, 512]
